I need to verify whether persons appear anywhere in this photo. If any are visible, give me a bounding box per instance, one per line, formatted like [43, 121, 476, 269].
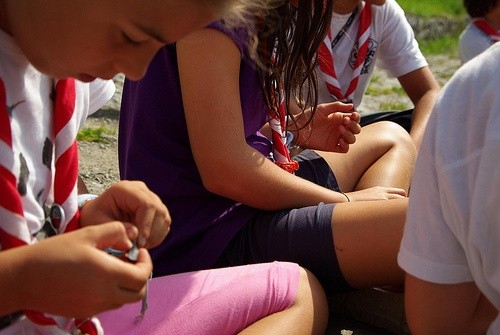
[273, 0, 441, 149]
[397, 42, 500, 335]
[0, 0, 327, 335]
[457, 0, 500, 63]
[118, 0, 418, 294]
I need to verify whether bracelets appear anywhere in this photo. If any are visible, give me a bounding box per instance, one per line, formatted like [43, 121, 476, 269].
[342, 192, 351, 202]
